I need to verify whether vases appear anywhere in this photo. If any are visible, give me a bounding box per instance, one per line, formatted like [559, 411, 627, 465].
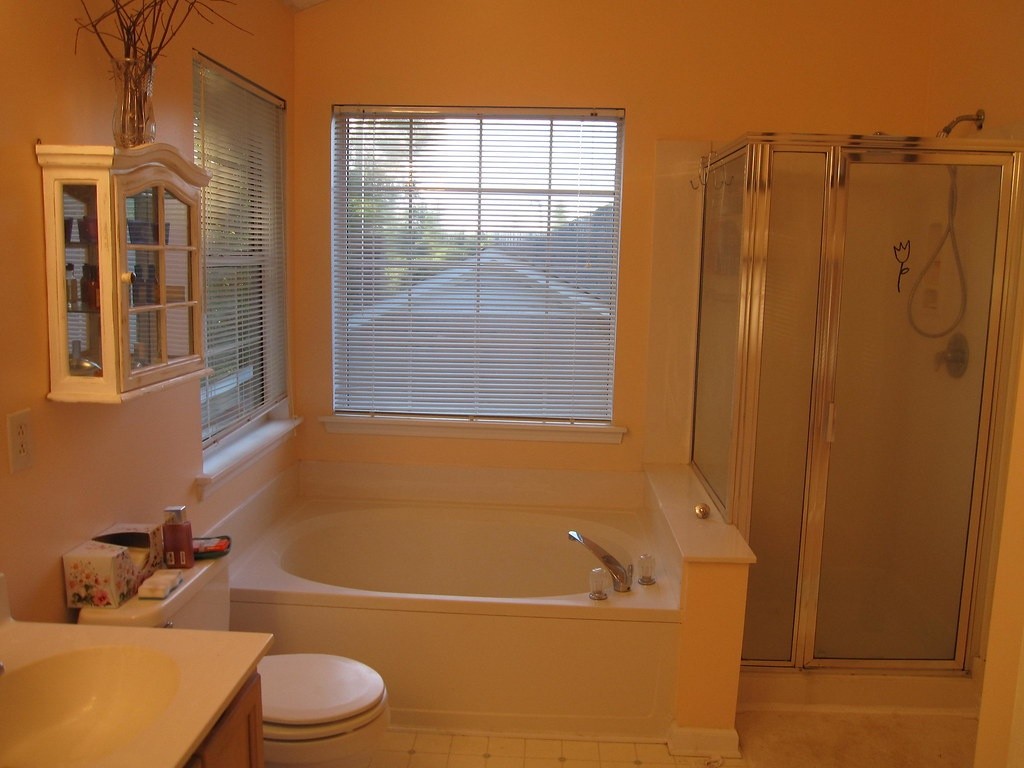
[111, 58, 157, 149]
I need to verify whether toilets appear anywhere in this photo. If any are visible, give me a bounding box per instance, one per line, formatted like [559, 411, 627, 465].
[77, 557, 392, 768]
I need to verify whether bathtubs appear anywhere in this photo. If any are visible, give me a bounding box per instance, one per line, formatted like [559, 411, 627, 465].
[228, 500, 680, 745]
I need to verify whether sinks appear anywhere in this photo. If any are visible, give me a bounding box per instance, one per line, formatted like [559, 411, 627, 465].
[1, 645, 180, 768]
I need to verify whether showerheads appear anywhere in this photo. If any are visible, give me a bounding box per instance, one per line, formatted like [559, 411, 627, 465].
[872, 131, 889, 136]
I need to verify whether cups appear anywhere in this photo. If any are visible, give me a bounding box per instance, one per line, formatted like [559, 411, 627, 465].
[77, 218, 98, 244]
[64, 215, 73, 243]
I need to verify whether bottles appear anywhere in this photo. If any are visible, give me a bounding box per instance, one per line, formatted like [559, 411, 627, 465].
[66, 263, 77, 303]
[80, 266, 91, 302]
[146, 265, 159, 303]
[132, 264, 146, 306]
[88, 265, 100, 310]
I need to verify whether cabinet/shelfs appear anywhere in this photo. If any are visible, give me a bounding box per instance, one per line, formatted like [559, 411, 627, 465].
[183, 665, 266, 768]
[35, 142, 212, 403]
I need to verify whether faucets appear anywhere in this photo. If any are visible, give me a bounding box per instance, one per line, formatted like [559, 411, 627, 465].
[568, 531, 633, 592]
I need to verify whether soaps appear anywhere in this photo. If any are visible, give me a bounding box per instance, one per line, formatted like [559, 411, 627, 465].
[138, 570, 183, 599]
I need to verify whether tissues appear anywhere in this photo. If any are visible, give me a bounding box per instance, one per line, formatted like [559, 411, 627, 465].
[62, 521, 164, 610]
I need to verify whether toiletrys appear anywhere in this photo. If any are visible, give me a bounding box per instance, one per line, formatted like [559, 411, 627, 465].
[161, 506, 194, 569]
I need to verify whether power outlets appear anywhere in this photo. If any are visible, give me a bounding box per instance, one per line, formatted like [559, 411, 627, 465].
[7, 408, 32, 474]
